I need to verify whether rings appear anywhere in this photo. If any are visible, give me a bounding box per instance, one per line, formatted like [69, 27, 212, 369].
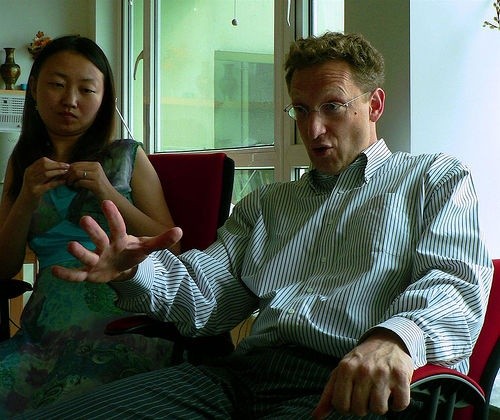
[83, 171, 86, 177]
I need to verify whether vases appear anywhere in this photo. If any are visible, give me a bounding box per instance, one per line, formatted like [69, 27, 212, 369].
[0, 47, 22, 90]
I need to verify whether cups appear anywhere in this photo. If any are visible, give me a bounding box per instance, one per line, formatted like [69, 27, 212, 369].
[21, 84, 27, 90]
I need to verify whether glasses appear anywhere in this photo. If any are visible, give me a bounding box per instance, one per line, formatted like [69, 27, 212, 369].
[283, 90, 375, 122]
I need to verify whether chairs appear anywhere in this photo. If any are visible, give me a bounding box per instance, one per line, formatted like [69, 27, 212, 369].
[393, 257, 500, 420]
[0, 152, 236, 362]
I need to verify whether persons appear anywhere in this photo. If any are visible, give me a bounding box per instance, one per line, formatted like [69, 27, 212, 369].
[0, 35, 182, 420]
[21, 34, 495, 420]
